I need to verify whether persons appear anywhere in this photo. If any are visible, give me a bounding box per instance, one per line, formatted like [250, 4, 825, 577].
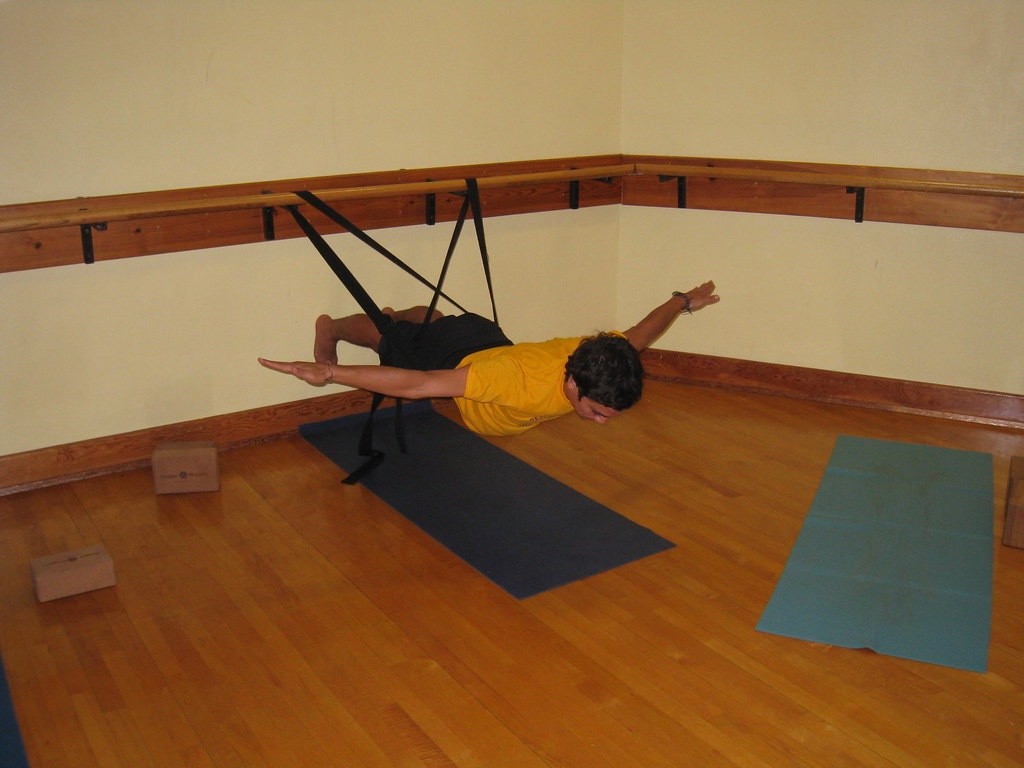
[259, 280, 720, 437]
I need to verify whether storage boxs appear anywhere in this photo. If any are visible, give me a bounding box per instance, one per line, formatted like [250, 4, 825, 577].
[1002, 456, 1024, 550]
[151, 441, 221, 494]
[28, 543, 116, 603]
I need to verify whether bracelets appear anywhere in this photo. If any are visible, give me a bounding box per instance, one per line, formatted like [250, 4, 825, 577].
[672, 291, 689, 308]
[327, 361, 334, 380]
